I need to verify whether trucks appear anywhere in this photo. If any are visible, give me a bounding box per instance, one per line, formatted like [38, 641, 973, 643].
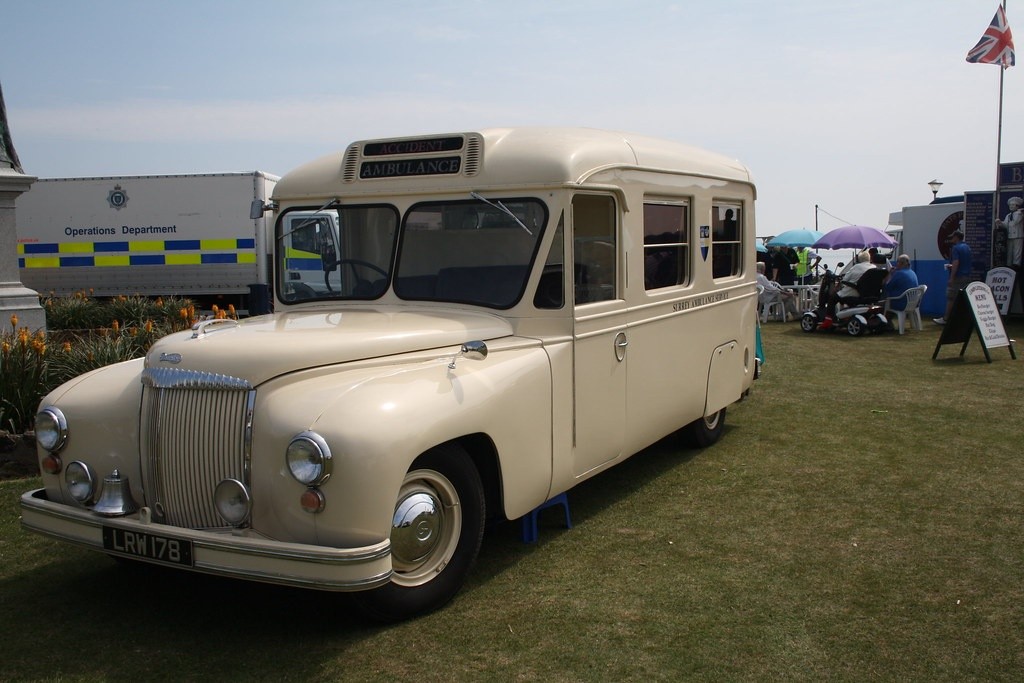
[15, 171, 344, 315]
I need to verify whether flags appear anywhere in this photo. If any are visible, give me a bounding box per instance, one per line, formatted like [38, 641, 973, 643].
[965, 2, 1016, 70]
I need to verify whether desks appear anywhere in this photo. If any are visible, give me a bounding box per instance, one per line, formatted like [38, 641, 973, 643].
[782, 285, 821, 319]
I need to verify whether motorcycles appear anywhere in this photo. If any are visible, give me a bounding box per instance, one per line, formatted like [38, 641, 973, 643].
[800, 262, 889, 337]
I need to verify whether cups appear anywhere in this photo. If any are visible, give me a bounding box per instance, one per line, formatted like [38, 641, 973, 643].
[794, 281, 798, 286]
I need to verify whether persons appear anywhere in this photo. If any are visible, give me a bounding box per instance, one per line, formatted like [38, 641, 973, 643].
[718, 209, 736, 241]
[462, 210, 516, 230]
[869, 248, 878, 263]
[757, 246, 822, 294]
[932, 231, 973, 325]
[878, 254, 919, 332]
[994, 196, 1024, 269]
[756, 261, 804, 321]
[644, 231, 683, 290]
[830, 251, 878, 323]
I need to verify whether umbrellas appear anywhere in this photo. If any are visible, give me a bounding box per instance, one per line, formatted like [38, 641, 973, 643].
[755, 239, 768, 253]
[812, 225, 900, 264]
[766, 227, 824, 248]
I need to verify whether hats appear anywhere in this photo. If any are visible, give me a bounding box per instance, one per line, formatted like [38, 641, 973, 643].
[948, 231, 965, 240]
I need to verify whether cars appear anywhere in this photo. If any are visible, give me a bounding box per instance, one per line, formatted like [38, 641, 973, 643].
[21, 125, 764, 615]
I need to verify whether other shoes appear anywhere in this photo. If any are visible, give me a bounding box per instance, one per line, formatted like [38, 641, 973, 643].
[934, 318, 948, 325]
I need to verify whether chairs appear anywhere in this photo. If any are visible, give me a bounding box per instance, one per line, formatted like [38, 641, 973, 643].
[808, 284, 819, 310]
[884, 284, 928, 335]
[757, 281, 789, 323]
[844, 268, 888, 307]
